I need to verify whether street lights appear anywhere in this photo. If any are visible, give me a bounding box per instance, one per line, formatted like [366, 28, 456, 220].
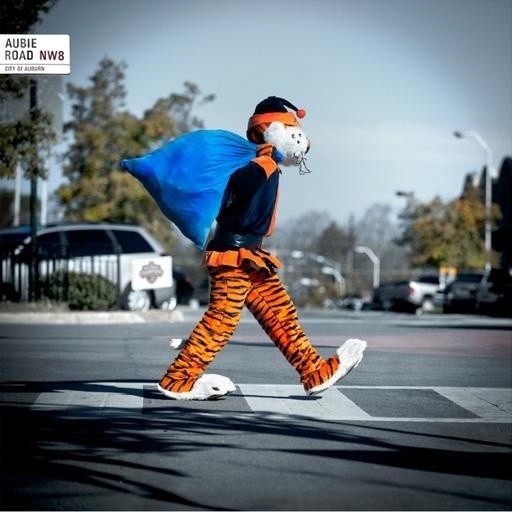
[453, 128, 493, 270]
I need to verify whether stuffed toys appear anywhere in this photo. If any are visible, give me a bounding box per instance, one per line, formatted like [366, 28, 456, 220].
[152, 94, 370, 405]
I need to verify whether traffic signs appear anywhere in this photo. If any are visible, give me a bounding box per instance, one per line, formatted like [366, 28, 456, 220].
[0, 33, 72, 77]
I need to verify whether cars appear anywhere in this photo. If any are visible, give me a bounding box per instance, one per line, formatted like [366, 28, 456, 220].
[336, 266, 511, 314]
[1, 221, 194, 314]
